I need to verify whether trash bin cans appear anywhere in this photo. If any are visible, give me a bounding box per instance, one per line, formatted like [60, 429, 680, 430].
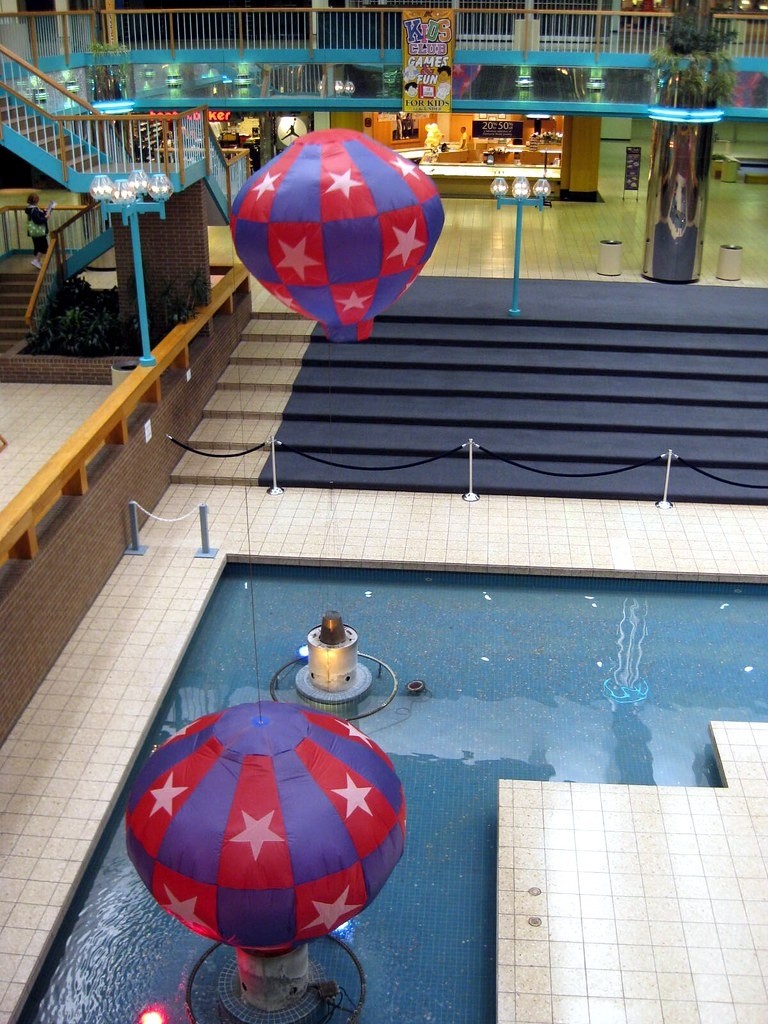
[716, 245, 743, 281]
[596, 239, 623, 276]
[111, 360, 140, 392]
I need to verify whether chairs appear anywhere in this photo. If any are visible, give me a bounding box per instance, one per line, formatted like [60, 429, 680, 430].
[513, 138, 523, 153]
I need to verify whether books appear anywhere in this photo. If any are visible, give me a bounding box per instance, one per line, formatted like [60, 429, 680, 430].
[46, 200, 56, 215]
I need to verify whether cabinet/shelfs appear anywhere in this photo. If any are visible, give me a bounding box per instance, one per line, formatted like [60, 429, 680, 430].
[139, 122, 162, 149]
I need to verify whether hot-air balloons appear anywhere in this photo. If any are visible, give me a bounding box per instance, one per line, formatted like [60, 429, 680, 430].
[124, 699, 410, 950]
[228, 127, 444, 341]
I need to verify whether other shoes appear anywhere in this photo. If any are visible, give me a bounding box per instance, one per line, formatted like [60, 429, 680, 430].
[31, 258, 42, 270]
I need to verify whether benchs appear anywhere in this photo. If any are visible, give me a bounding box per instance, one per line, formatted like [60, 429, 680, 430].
[744, 173, 768, 184]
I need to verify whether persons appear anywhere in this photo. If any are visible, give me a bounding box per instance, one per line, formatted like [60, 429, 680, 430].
[652, 180, 675, 281]
[234, 123, 240, 144]
[621, 0, 636, 28]
[640, 0, 654, 28]
[24, 192, 51, 270]
[395, 113, 403, 140]
[458, 127, 467, 150]
[675, 185, 698, 280]
[401, 113, 414, 139]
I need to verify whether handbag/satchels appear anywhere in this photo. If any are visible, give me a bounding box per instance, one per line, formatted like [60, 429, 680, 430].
[28, 207, 46, 237]
